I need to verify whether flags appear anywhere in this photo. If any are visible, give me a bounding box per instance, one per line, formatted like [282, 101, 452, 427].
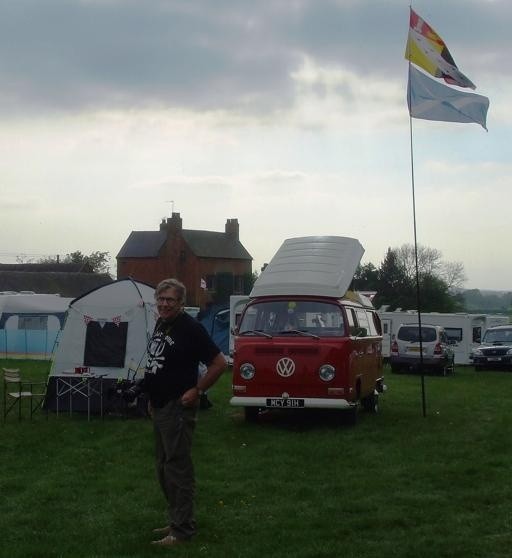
[407, 63, 490, 132]
[405, 4, 478, 90]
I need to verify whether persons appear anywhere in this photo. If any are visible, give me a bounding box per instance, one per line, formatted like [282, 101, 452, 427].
[142, 277, 228, 549]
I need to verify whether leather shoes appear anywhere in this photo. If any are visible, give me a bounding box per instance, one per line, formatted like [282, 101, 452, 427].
[151, 525, 184, 546]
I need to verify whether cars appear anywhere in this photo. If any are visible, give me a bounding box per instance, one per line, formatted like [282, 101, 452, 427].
[469, 324, 512, 371]
[389, 324, 456, 377]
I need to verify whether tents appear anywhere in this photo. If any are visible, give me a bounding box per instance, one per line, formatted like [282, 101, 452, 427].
[0, 291, 77, 363]
[40, 273, 215, 414]
[198, 295, 249, 366]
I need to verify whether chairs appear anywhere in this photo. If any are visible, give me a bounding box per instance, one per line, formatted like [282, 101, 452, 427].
[2, 367, 33, 423]
[103, 369, 137, 421]
[443, 352, 455, 377]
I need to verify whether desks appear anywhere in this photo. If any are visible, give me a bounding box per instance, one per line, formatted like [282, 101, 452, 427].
[49, 373, 107, 423]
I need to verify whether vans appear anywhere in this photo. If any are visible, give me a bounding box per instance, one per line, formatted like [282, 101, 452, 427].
[229, 238, 386, 429]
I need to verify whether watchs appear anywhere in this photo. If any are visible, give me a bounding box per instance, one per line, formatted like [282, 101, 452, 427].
[195, 386, 204, 399]
